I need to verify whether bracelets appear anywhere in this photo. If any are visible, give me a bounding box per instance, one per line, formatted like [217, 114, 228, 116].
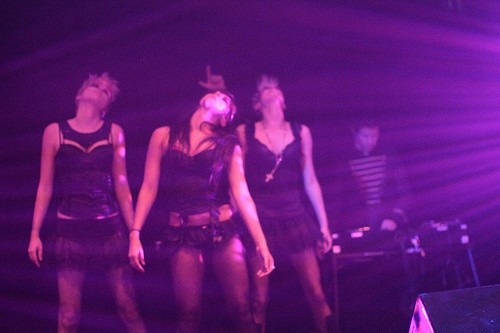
[128, 229, 141, 232]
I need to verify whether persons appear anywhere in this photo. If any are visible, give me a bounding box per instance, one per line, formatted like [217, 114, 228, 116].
[235, 72, 333, 333]
[329, 114, 406, 238]
[197, 64, 239, 105]
[129, 90, 276, 333]
[28, 72, 142, 333]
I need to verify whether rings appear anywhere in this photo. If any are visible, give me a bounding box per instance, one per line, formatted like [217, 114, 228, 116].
[270, 265, 275, 269]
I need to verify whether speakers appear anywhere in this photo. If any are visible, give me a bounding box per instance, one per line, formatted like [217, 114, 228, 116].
[316, 242, 480, 333]
[409, 284, 500, 333]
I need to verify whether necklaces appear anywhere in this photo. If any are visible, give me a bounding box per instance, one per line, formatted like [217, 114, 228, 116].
[261, 119, 287, 154]
